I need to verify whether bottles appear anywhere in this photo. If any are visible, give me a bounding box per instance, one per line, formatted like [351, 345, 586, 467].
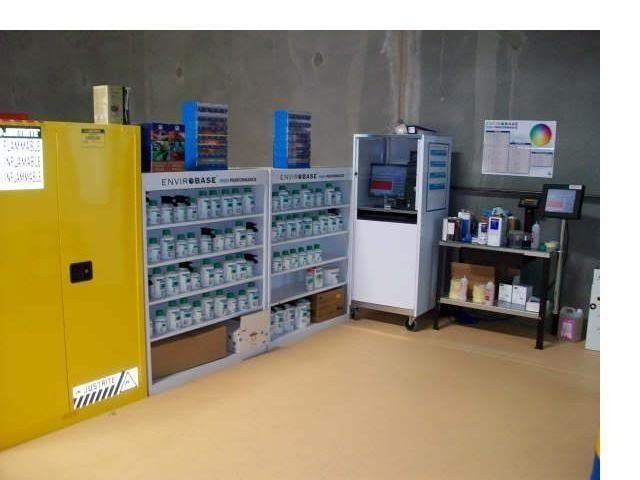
[484, 280, 496, 306]
[458, 274, 469, 302]
[144, 181, 344, 336]
[530, 219, 541, 249]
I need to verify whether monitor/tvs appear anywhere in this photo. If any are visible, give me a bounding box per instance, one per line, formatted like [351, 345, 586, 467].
[537, 183, 585, 219]
[368, 162, 408, 200]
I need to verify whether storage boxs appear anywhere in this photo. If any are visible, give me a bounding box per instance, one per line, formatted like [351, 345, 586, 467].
[224, 311, 268, 354]
[151, 323, 228, 380]
[584, 268, 600, 353]
[309, 288, 345, 322]
[137, 122, 185, 172]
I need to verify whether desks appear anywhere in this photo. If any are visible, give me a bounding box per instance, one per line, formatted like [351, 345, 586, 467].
[431, 238, 567, 350]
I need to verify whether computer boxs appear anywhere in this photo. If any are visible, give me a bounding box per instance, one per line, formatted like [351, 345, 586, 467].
[406, 161, 417, 210]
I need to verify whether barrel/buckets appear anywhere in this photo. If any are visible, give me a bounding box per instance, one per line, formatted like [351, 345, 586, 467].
[544, 300, 558, 336]
[557, 306, 584, 343]
[323, 266, 340, 287]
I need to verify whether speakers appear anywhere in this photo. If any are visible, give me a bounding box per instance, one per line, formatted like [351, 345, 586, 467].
[410, 151, 417, 161]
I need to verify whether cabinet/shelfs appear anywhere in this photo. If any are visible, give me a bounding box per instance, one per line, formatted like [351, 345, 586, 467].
[268, 166, 353, 351]
[139, 167, 268, 396]
[347, 132, 453, 330]
[0, 119, 149, 452]
